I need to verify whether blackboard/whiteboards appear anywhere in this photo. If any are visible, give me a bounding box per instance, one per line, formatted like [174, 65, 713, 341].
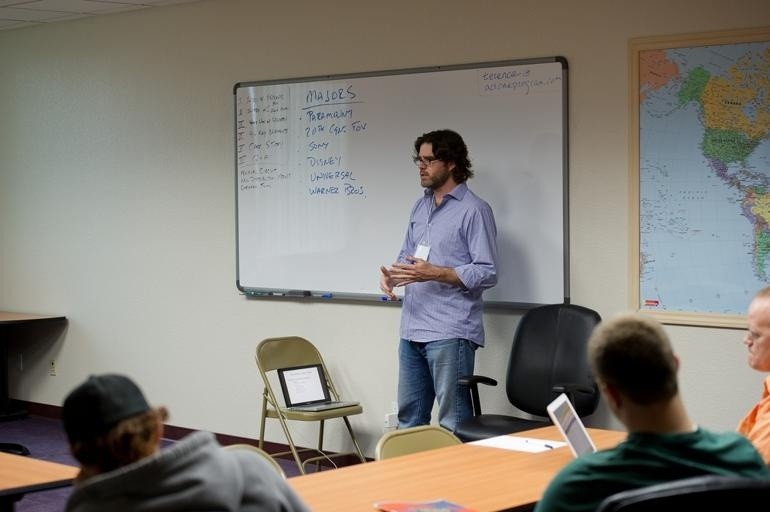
[233, 55, 571, 312]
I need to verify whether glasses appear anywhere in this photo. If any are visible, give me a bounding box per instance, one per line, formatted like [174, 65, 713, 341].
[413, 155, 443, 168]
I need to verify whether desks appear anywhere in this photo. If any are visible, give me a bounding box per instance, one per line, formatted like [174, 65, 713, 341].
[282, 425, 628, 512]
[1, 306, 67, 423]
[0, 444, 82, 512]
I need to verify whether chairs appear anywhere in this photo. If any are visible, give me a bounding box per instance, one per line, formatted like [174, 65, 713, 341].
[254, 336, 367, 475]
[596, 477, 767, 512]
[454, 303, 604, 442]
[374, 426, 463, 461]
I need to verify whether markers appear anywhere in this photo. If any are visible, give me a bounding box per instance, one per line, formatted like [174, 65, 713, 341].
[383, 297, 401, 301]
[269, 292, 285, 295]
[288, 291, 310, 295]
[313, 293, 332, 297]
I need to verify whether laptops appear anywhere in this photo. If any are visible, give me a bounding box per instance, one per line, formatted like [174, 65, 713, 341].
[547, 394, 598, 459]
[277, 363, 361, 413]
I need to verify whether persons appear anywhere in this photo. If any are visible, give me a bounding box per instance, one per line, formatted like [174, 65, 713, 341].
[734, 285, 770, 465]
[531, 311, 770, 511]
[59, 371, 310, 511]
[378, 128, 499, 434]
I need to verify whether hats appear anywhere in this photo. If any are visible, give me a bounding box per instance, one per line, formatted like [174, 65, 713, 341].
[61, 373, 153, 440]
